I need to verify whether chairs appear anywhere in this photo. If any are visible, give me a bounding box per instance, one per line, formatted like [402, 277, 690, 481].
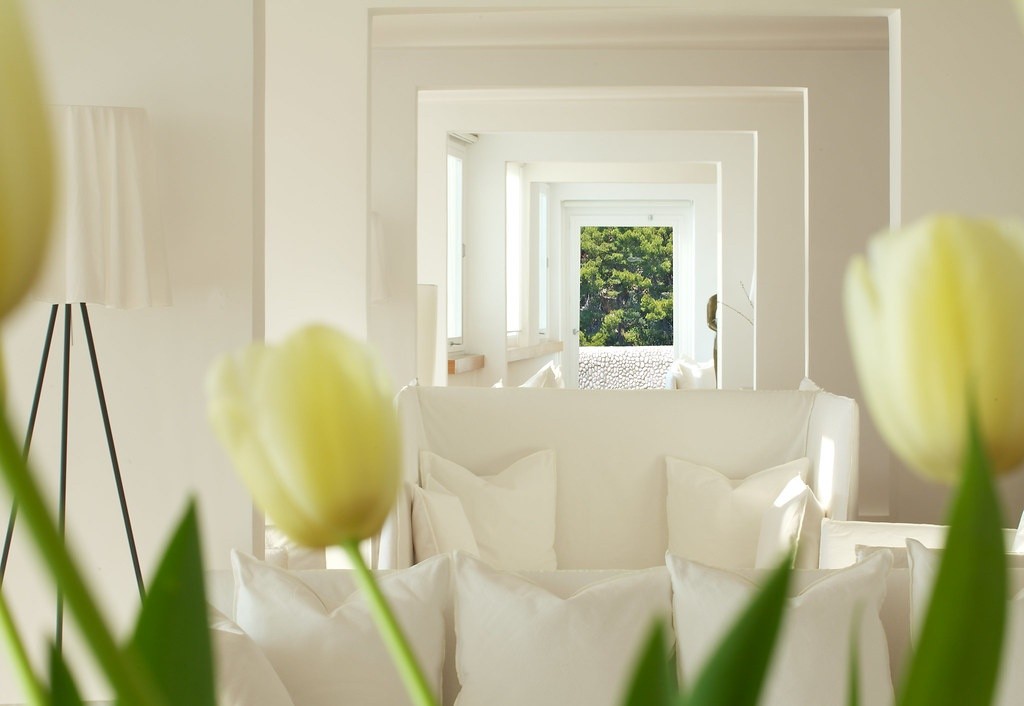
[821, 505, 1024, 706]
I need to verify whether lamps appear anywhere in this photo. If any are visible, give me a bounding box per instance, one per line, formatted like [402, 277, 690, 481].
[0, 103, 160, 656]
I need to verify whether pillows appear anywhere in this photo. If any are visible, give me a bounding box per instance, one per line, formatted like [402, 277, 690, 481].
[754, 471, 824, 566]
[412, 485, 481, 566]
[664, 457, 812, 566]
[906, 538, 952, 658]
[664, 548, 902, 706]
[229, 548, 452, 706]
[418, 446, 563, 571]
[450, 547, 677, 706]
[490, 360, 575, 389]
[205, 599, 296, 706]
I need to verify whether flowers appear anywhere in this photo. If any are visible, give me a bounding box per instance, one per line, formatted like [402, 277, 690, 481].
[0, 0, 1024, 706]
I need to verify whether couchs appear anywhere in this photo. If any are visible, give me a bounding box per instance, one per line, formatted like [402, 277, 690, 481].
[377, 384, 860, 571]
[667, 350, 719, 390]
